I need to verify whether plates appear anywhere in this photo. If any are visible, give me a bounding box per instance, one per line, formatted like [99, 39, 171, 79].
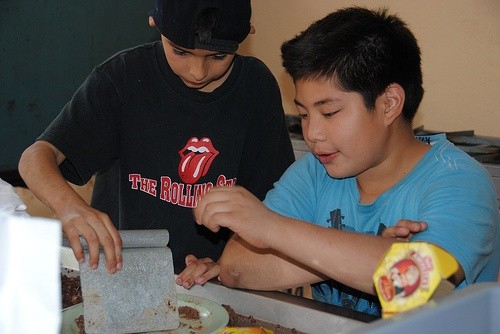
[61, 292, 228, 333]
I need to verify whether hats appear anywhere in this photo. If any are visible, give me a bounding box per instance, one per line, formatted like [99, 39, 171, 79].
[149, 1, 252, 52]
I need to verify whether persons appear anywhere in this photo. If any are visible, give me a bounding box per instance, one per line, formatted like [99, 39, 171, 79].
[194, 4, 499, 318]
[18, 0, 296, 288]
